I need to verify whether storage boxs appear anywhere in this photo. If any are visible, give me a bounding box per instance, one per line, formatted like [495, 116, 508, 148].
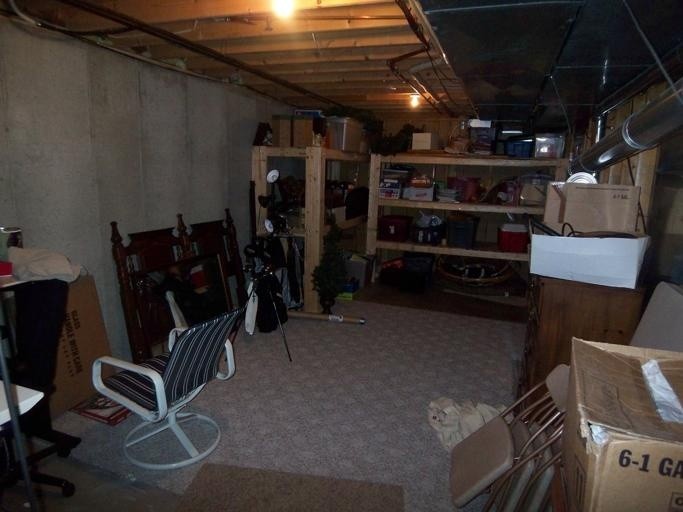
[527, 220, 653, 292]
[495, 129, 565, 159]
[270, 108, 370, 153]
[544, 182, 641, 236]
[561, 332, 682, 511]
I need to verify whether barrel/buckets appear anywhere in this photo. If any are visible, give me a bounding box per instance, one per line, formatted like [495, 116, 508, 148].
[447, 214, 481, 249]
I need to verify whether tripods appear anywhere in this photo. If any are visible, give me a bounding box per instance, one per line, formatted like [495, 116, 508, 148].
[222, 263, 292, 361]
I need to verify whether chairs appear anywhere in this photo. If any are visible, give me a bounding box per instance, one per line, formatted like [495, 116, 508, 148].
[444, 360, 573, 511]
[89, 306, 247, 469]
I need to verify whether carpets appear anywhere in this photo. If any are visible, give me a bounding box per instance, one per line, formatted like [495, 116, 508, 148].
[173, 461, 404, 510]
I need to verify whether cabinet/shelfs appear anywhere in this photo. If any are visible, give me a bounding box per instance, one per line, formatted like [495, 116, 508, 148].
[367, 153, 569, 299]
[252, 146, 367, 320]
